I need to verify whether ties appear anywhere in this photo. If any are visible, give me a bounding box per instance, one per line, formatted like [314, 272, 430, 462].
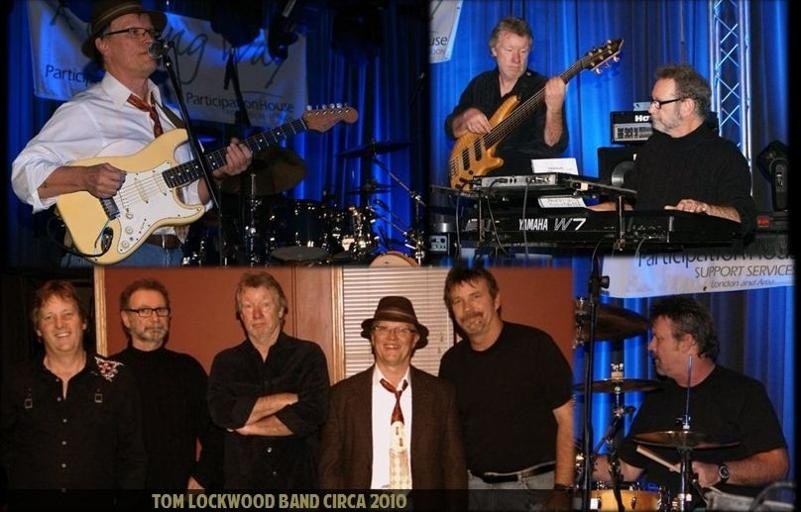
[380, 379, 413, 499]
[127, 92, 190, 243]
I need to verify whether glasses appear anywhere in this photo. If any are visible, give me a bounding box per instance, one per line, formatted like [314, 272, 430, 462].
[124, 306, 171, 317]
[647, 96, 683, 109]
[105, 27, 163, 39]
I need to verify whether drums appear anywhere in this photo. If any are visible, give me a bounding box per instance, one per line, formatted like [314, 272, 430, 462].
[331, 210, 376, 259]
[346, 250, 420, 268]
[269, 200, 333, 265]
[586, 481, 670, 510]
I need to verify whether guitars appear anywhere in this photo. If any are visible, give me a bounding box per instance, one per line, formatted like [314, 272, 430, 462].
[53, 103, 358, 264]
[450, 40, 624, 191]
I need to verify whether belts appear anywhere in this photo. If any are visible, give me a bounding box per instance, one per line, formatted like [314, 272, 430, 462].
[144, 234, 183, 248]
[471, 463, 557, 483]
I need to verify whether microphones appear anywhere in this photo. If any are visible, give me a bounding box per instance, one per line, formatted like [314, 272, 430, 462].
[148, 41, 174, 60]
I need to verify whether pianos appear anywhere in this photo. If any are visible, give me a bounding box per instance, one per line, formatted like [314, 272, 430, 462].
[480, 211, 742, 248]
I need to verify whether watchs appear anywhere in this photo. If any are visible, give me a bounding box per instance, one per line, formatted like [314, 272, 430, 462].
[554, 482, 578, 498]
[716, 460, 730, 488]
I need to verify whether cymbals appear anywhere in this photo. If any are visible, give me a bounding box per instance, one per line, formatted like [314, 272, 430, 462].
[634, 430, 740, 450]
[336, 141, 409, 158]
[218, 147, 307, 196]
[574, 301, 653, 341]
[572, 379, 664, 392]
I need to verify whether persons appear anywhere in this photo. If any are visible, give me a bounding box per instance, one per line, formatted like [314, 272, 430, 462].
[309, 295, 469, 511]
[208, 269, 331, 511]
[437, 266, 578, 511]
[593, 294, 792, 510]
[445, 17, 570, 203]
[586, 61, 755, 250]
[105, 276, 217, 512]
[1, 281, 147, 512]
[11, 0, 253, 272]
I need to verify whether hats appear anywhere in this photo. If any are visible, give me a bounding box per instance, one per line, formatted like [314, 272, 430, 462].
[80, 0, 167, 59]
[361, 296, 428, 349]
